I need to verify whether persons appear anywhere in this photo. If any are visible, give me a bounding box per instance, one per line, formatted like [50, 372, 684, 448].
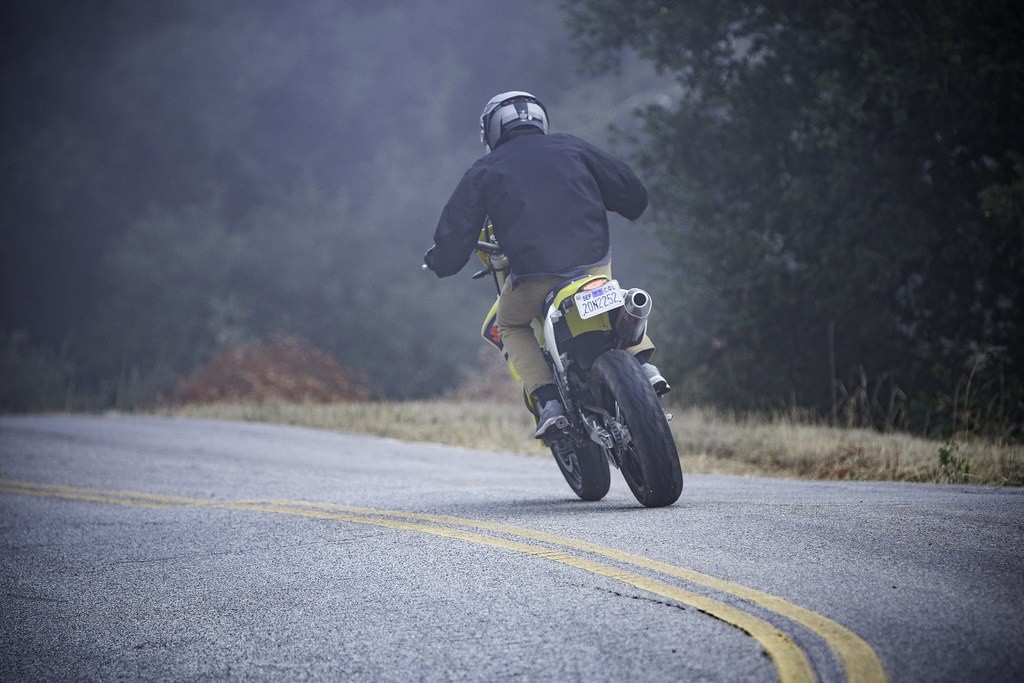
[423, 91, 672, 439]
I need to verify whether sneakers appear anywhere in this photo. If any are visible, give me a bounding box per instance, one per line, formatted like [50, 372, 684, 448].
[641, 361, 671, 396]
[534, 393, 568, 438]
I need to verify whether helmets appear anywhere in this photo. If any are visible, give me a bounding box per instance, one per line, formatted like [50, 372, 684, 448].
[480, 90, 549, 152]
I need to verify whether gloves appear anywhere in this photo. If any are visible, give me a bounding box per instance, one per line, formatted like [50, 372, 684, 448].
[424, 245, 435, 268]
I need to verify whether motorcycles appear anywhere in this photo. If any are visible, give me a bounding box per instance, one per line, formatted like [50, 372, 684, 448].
[421, 213, 682, 508]
